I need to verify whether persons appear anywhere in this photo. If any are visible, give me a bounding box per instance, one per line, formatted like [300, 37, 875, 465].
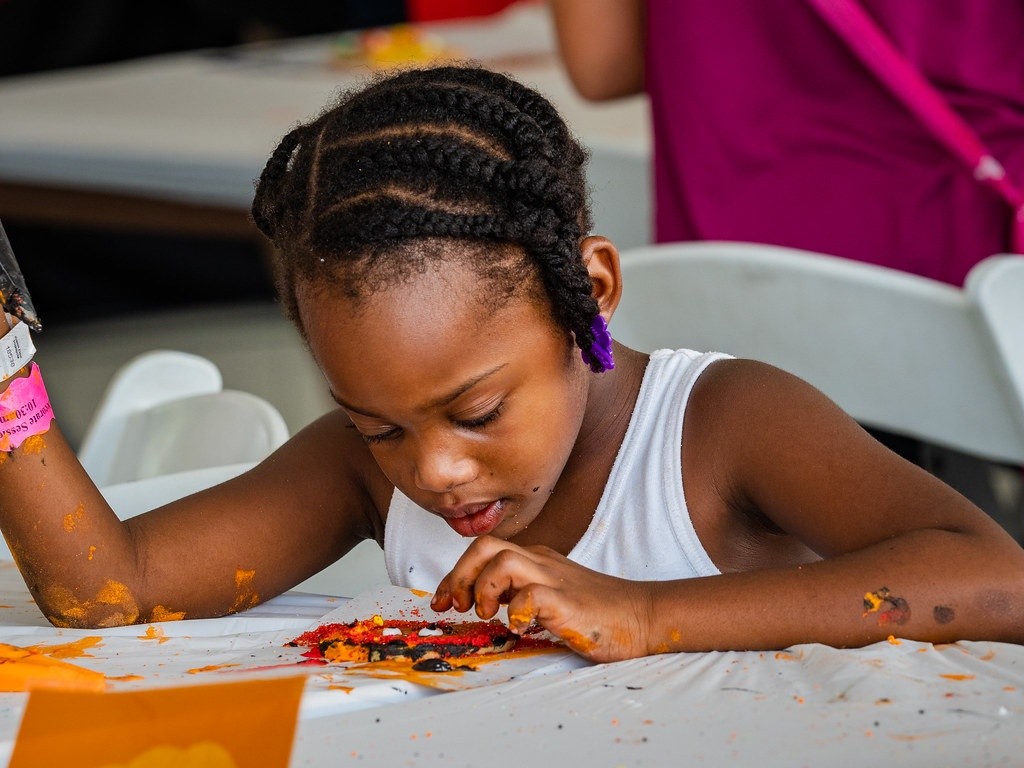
[0, 64, 1024, 663]
[552, 1, 1023, 552]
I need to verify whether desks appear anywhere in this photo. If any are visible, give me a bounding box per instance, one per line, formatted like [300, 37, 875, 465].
[0, 557, 1024, 768]
[0, 23, 651, 244]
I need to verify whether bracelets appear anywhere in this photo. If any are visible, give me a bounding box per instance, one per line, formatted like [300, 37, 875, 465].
[1, 315, 56, 455]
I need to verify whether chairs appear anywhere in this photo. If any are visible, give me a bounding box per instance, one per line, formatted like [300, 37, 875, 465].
[607, 241, 1024, 466]
